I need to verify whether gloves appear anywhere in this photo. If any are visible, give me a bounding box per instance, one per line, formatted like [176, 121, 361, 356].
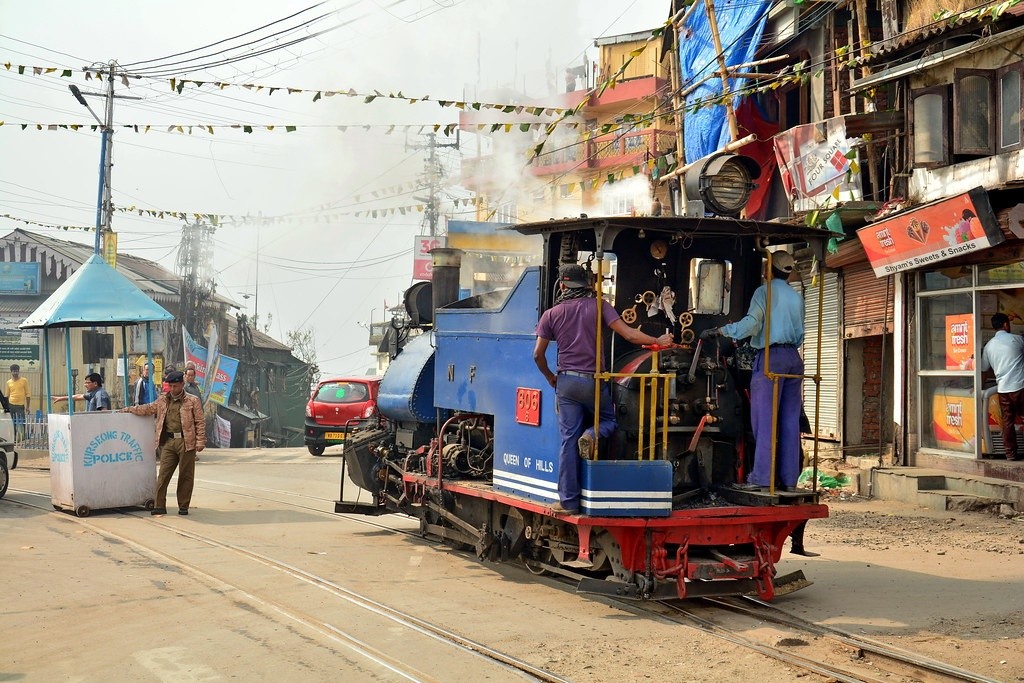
[700, 326, 721, 342]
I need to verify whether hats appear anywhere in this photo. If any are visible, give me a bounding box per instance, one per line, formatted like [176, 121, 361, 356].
[165, 371, 184, 383]
[771, 250, 794, 273]
[559, 264, 588, 288]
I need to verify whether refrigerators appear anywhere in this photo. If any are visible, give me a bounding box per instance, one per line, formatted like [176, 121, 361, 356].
[933, 378, 1024, 460]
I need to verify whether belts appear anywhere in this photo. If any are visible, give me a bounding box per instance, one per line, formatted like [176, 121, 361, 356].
[558, 370, 593, 379]
[167, 431, 184, 438]
[772, 342, 795, 347]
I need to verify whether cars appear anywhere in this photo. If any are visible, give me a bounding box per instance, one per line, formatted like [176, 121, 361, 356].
[303, 376, 383, 456]
[0, 390, 18, 499]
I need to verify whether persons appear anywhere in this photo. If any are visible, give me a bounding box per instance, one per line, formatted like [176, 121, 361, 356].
[117, 362, 206, 515]
[533, 263, 674, 515]
[981, 313, 1024, 460]
[700, 250, 805, 492]
[4, 364, 31, 444]
[50, 373, 112, 411]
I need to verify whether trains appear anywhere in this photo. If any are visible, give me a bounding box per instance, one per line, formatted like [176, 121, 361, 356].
[333, 218, 846, 599]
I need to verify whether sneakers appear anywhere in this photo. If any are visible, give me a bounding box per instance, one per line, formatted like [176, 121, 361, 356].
[578, 434, 594, 460]
[550, 502, 580, 514]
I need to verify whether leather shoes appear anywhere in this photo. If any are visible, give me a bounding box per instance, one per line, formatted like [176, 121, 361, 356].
[732, 481, 769, 492]
[778, 482, 796, 492]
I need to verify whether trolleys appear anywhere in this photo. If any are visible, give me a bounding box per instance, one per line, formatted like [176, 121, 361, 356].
[47, 411, 157, 517]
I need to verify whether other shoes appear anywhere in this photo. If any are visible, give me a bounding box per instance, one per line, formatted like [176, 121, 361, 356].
[194, 455, 199, 461]
[1008, 456, 1016, 461]
[179, 508, 188, 515]
[151, 508, 167, 515]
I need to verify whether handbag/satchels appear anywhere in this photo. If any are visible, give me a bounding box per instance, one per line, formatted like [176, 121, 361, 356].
[669, 448, 703, 503]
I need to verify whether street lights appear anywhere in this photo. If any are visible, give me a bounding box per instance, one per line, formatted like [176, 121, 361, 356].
[69, 85, 106, 255]
